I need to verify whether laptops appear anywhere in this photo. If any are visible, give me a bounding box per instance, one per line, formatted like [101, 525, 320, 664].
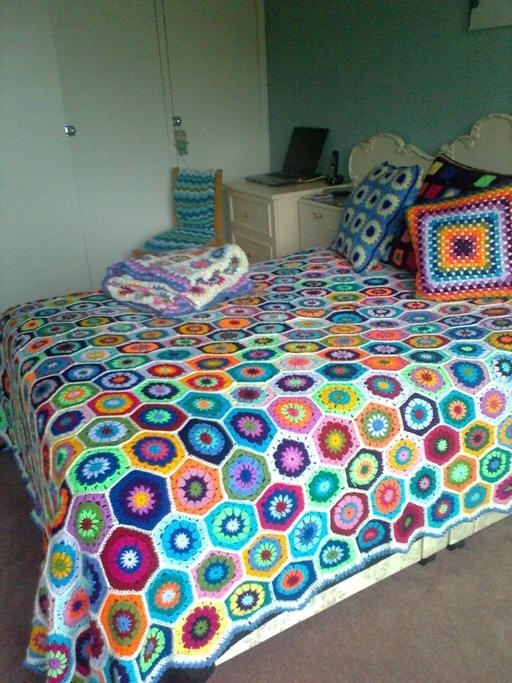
[246, 127, 328, 187]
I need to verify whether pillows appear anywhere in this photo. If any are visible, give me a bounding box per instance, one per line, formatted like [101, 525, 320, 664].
[330, 151, 512, 303]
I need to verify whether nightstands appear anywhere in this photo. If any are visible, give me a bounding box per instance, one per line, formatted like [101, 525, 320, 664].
[220, 173, 350, 263]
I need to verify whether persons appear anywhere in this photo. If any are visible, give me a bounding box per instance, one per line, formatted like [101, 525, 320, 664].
[326, 164, 341, 183]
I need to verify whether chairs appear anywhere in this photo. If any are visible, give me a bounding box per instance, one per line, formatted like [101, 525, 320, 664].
[131, 166, 224, 259]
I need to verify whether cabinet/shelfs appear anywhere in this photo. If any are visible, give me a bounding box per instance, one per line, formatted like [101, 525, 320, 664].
[0, 0, 272, 314]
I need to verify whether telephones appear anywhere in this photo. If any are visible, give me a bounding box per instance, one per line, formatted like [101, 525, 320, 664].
[326, 150, 343, 184]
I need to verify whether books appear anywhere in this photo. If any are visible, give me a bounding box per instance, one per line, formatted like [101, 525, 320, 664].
[305, 184, 352, 207]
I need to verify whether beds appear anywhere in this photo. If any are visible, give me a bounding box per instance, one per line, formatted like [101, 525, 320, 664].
[1, 112, 512, 683]
